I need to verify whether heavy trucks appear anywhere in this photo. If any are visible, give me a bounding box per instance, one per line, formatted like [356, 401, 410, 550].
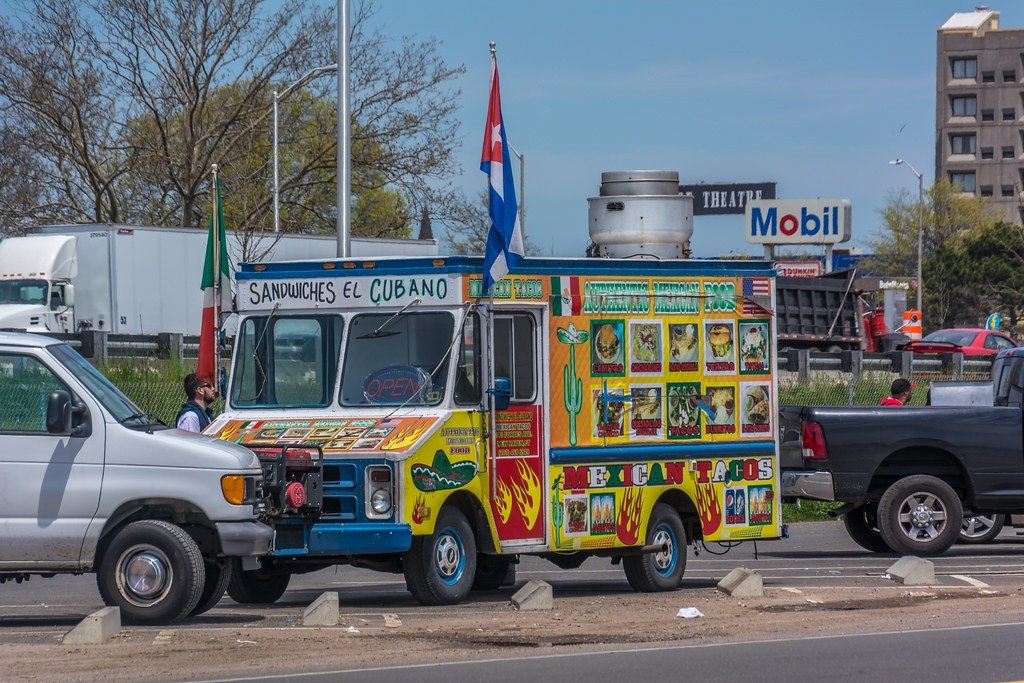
[1, 226, 442, 347]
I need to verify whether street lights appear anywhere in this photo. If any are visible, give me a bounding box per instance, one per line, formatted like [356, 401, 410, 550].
[271, 63, 338, 232]
[887, 153, 927, 329]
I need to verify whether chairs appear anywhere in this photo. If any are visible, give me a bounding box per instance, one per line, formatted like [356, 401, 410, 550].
[429, 363, 478, 403]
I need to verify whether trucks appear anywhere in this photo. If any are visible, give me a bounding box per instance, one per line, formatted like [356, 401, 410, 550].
[201, 173, 783, 604]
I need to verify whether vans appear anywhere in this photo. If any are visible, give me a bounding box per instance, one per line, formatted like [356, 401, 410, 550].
[1, 330, 276, 624]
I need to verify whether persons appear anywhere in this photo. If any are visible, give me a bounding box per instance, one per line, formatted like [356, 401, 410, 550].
[175, 374, 215, 434]
[881, 379, 916, 406]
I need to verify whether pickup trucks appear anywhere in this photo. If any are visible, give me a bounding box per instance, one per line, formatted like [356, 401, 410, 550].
[779, 346, 1024, 556]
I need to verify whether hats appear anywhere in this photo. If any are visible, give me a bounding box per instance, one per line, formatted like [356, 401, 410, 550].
[891, 379, 917, 394]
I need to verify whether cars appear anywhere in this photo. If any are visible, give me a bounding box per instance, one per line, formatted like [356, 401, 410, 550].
[905, 324, 1018, 357]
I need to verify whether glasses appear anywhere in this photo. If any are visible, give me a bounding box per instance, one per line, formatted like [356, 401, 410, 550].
[194, 383, 213, 391]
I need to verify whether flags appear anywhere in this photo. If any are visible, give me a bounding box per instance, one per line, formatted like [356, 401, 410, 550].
[196, 175, 232, 384]
[482, 60, 525, 296]
